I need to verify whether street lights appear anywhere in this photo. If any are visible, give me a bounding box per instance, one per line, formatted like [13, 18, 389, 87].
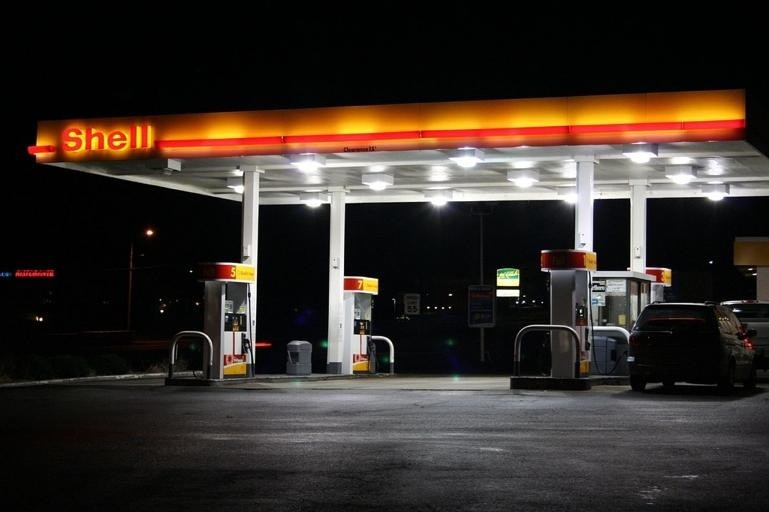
[126, 227, 157, 327]
[392, 298, 396, 320]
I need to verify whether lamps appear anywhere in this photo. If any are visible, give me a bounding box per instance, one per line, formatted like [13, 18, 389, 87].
[226, 177, 248, 194]
[299, 192, 327, 209]
[291, 154, 325, 175]
[423, 188, 463, 207]
[701, 185, 729, 201]
[665, 165, 697, 185]
[506, 170, 540, 189]
[622, 143, 659, 164]
[362, 174, 394, 191]
[449, 149, 484, 168]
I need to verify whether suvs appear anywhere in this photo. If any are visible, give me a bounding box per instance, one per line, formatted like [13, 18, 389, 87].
[718, 298, 768, 377]
[627, 301, 758, 392]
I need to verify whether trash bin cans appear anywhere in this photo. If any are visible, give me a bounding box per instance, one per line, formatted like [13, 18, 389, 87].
[286, 340, 312, 375]
[591, 336, 617, 374]
